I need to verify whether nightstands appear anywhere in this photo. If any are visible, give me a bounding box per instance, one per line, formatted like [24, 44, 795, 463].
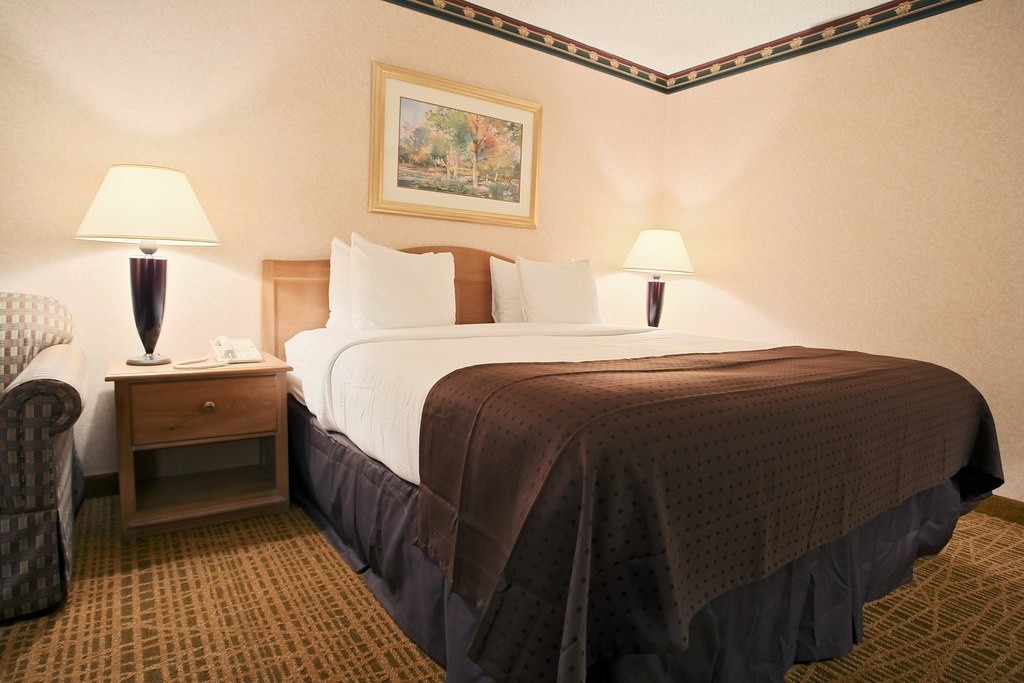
[104, 349, 295, 538]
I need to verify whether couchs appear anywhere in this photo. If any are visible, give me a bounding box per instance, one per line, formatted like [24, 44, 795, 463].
[0, 290, 86, 622]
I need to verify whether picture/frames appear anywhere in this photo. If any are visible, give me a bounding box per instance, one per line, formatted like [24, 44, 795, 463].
[368, 61, 543, 229]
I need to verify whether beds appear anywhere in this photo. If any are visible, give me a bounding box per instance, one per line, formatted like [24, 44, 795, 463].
[263, 247, 1004, 683]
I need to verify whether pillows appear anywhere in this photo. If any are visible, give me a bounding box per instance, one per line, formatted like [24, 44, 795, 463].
[515, 254, 601, 323]
[325, 237, 435, 328]
[350, 231, 456, 328]
[489, 256, 527, 323]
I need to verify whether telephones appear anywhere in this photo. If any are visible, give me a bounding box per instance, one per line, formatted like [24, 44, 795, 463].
[210, 336, 263, 364]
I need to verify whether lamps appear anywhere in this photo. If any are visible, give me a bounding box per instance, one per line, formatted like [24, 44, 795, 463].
[75, 164, 219, 367]
[621, 230, 696, 327]
